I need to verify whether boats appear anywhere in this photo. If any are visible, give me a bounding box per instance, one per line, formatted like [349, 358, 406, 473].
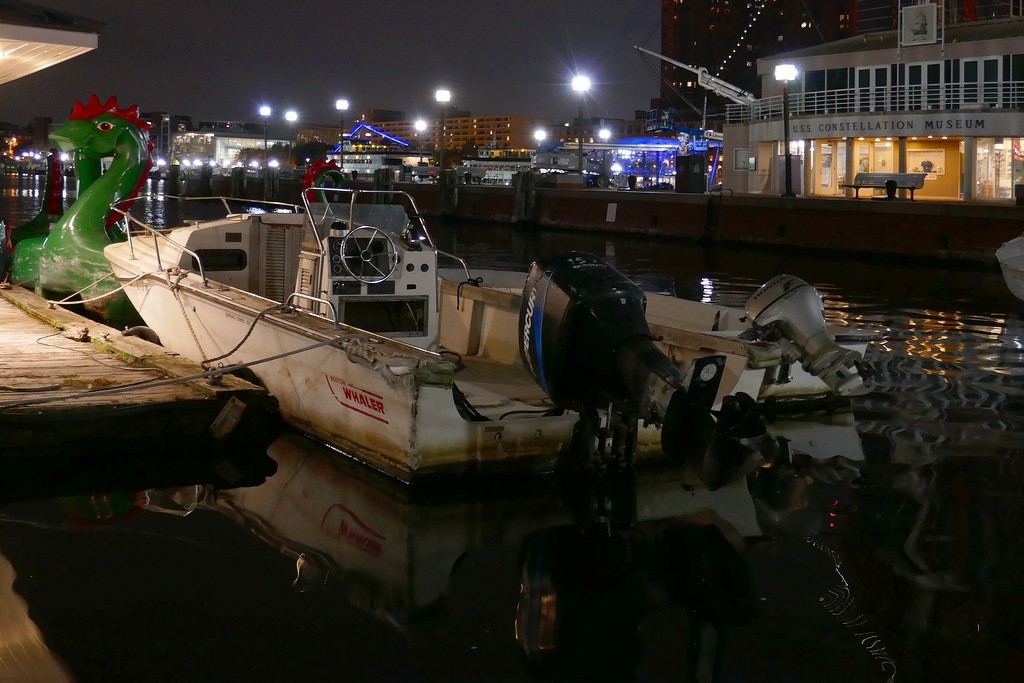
[7, 89, 303, 323]
[104, 180, 882, 492]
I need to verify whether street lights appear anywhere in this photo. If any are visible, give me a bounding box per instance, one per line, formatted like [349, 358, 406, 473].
[535, 128, 547, 154]
[775, 65, 800, 197]
[260, 105, 272, 177]
[285, 110, 297, 165]
[335, 97, 349, 171]
[571, 74, 592, 187]
[598, 128, 613, 187]
[434, 88, 451, 187]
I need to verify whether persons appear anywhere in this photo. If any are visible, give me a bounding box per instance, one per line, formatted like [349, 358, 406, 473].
[921, 160, 932, 172]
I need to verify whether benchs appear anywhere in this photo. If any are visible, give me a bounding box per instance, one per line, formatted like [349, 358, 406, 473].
[843, 172, 927, 202]
